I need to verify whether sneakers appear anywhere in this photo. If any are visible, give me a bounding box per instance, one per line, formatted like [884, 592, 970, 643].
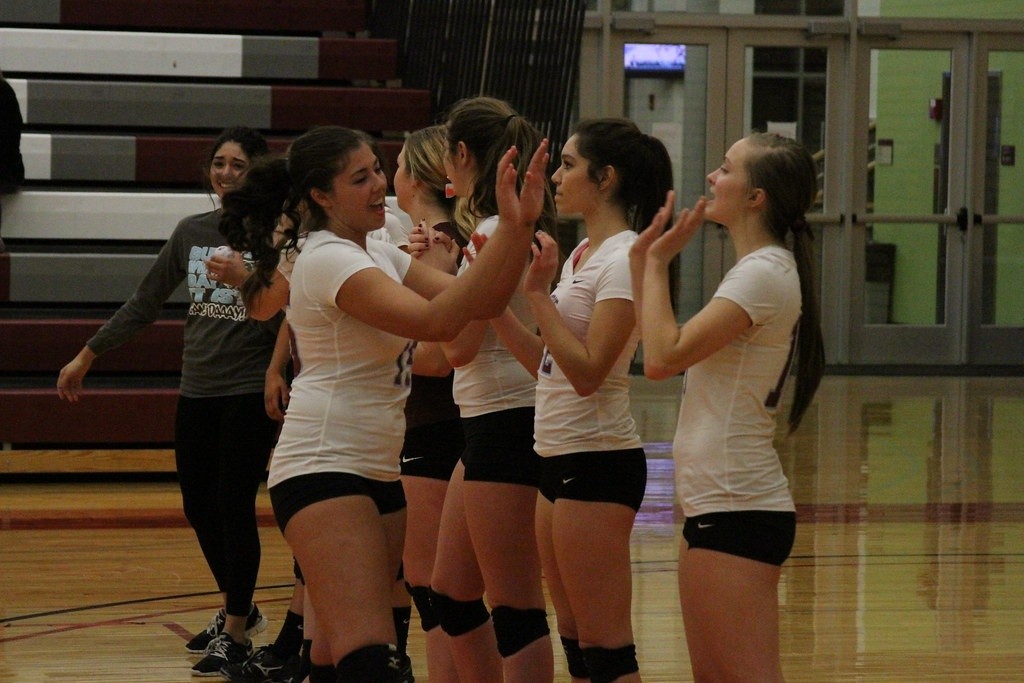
[220, 644, 300, 683]
[191, 631, 254, 676]
[185, 600, 268, 653]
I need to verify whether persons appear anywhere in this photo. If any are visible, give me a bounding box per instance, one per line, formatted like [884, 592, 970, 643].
[0, 68, 26, 252]
[629, 133, 827, 683]
[58, 98, 683, 683]
